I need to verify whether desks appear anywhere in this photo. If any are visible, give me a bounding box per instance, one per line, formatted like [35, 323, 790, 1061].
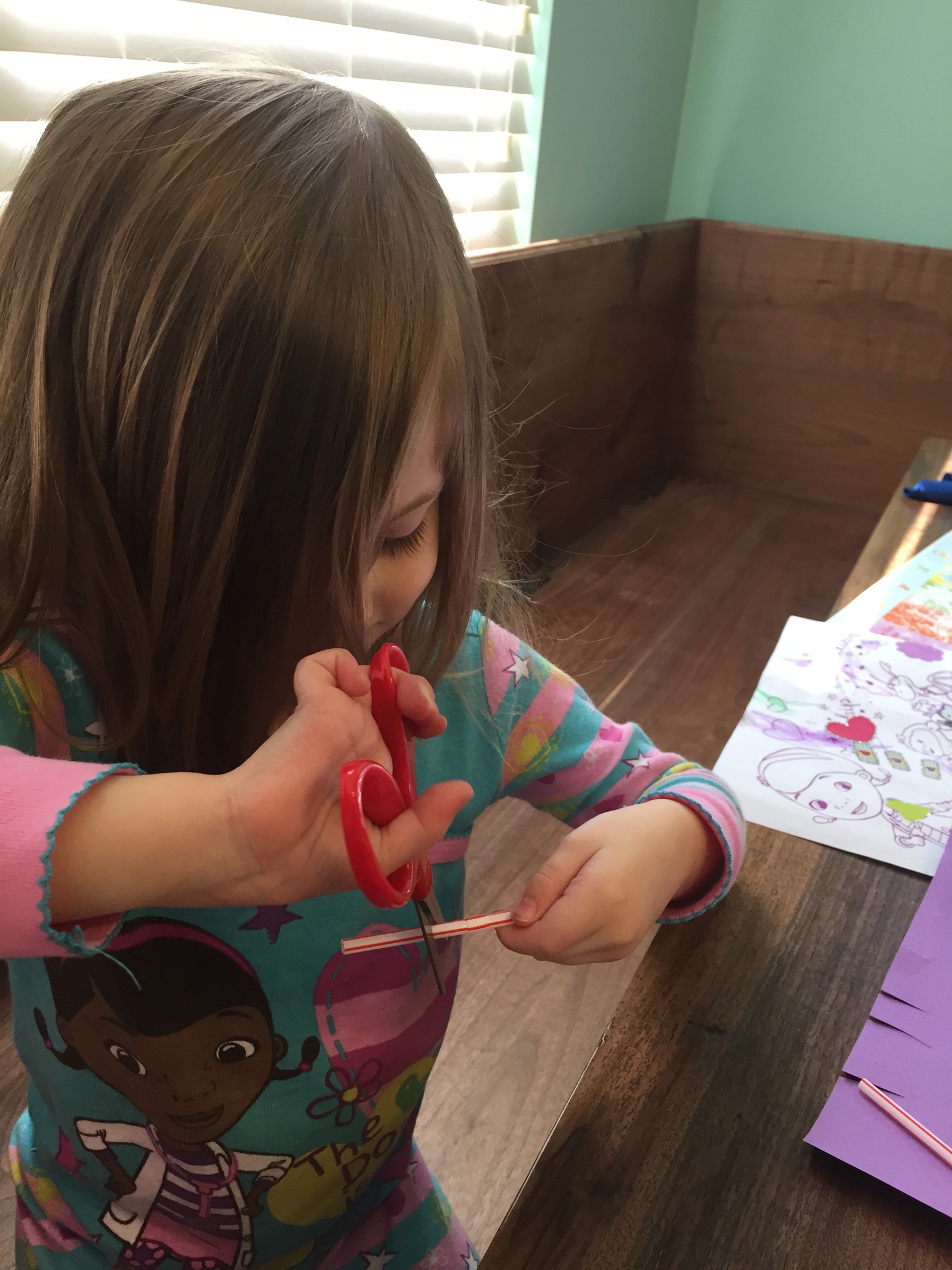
[471, 438, 952, 1269]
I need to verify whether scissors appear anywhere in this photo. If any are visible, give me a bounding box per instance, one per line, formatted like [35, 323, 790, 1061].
[338, 641, 452, 995]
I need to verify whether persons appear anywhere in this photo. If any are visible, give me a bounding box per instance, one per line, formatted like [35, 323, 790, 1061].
[0, 64, 752, 1269]
